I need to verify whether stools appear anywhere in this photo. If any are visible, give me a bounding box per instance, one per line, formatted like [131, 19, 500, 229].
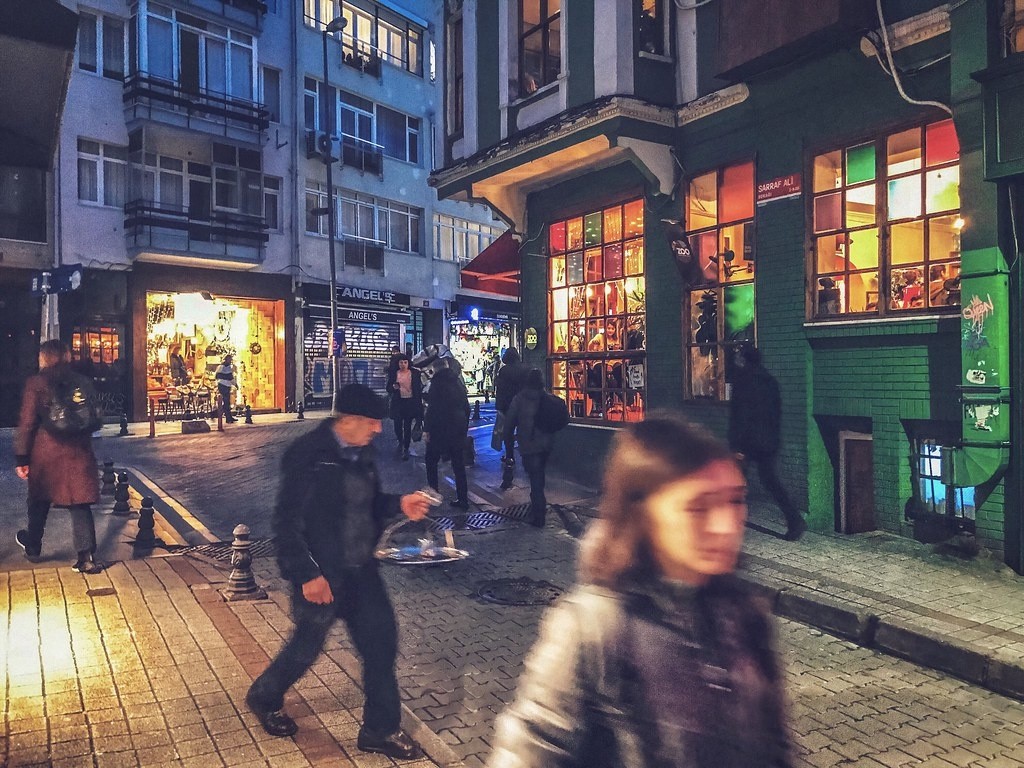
[154, 393, 215, 423]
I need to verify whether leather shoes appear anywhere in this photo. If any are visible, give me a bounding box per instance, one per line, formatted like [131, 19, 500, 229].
[357, 724, 421, 758]
[246, 691, 299, 736]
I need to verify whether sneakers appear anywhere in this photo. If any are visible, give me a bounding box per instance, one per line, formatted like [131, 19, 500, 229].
[71, 552, 95, 573]
[15, 530, 38, 555]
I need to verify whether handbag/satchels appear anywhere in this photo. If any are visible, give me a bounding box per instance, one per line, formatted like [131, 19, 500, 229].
[491, 411, 505, 451]
[411, 423, 423, 443]
[534, 396, 569, 434]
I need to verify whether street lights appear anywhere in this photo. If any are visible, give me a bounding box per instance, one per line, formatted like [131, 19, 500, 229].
[322, 16, 348, 418]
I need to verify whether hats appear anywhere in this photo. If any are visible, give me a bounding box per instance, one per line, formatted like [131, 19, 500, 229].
[336, 384, 389, 420]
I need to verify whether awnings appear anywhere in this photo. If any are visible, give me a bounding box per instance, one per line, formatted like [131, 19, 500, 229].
[461, 228, 521, 297]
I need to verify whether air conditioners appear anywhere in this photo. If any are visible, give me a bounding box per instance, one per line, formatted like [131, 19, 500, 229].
[307, 129, 340, 162]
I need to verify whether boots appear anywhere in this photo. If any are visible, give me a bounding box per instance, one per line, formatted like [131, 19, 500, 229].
[529, 490, 545, 527]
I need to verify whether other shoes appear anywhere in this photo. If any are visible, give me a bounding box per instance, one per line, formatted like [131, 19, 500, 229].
[397, 438, 403, 451]
[402, 448, 410, 460]
[784, 519, 808, 541]
[449, 497, 469, 509]
[226, 418, 238, 423]
[500, 455, 515, 465]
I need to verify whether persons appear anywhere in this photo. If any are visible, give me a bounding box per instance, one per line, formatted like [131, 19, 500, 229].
[12, 340, 98, 573]
[245, 384, 429, 759]
[473, 354, 501, 397]
[411, 344, 470, 512]
[383, 342, 420, 459]
[587, 310, 636, 418]
[501, 368, 557, 527]
[485, 418, 791, 768]
[209, 354, 238, 423]
[725, 343, 806, 541]
[889, 264, 946, 308]
[495, 345, 533, 461]
[170, 346, 187, 386]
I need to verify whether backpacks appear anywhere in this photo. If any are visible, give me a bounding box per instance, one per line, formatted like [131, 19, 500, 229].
[37, 369, 103, 443]
